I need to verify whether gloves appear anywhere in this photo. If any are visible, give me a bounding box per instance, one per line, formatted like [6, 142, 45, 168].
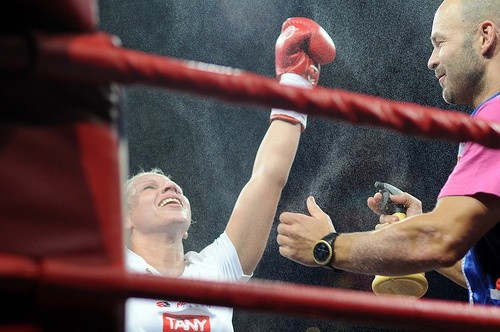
[269, 17, 337, 129]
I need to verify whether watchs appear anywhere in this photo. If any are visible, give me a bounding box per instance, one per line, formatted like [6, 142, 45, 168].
[312, 233, 339, 271]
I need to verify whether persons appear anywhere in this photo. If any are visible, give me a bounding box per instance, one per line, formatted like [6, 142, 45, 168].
[276, 0, 500, 305]
[122, 17, 337, 332]
